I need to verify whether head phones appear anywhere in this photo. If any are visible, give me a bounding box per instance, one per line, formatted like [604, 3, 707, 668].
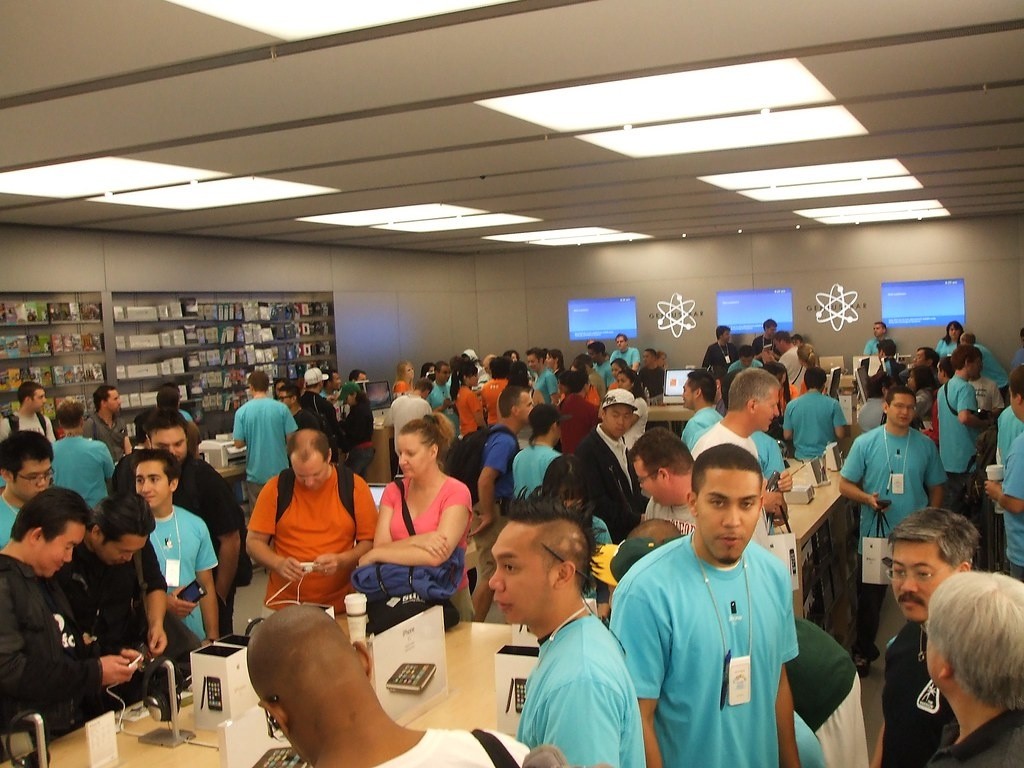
[7, 709, 51, 768]
[142, 656, 183, 722]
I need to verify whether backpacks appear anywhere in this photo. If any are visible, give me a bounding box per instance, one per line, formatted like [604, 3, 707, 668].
[445, 424, 520, 494]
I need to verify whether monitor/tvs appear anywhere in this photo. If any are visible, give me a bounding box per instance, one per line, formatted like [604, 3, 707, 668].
[819, 354, 882, 405]
[662, 369, 698, 404]
[363, 381, 392, 417]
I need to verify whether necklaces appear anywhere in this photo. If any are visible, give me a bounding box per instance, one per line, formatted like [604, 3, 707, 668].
[918, 627, 927, 662]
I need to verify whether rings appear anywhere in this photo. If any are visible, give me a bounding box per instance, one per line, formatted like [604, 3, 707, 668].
[325, 569, 327, 573]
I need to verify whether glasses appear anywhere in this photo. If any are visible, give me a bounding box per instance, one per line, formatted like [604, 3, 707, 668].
[16, 466, 55, 483]
[638, 465, 659, 483]
[263, 696, 285, 742]
[889, 564, 951, 582]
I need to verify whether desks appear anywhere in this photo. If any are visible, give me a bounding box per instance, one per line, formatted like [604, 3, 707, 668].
[647, 405, 696, 431]
[366, 419, 403, 483]
[766, 450, 854, 650]
[0, 611, 539, 768]
[825, 374, 856, 387]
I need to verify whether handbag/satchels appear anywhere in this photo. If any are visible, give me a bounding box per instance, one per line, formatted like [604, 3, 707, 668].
[366, 563, 460, 635]
[767, 505, 799, 590]
[862, 509, 893, 584]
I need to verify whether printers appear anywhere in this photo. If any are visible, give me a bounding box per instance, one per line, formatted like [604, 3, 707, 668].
[198, 438, 249, 467]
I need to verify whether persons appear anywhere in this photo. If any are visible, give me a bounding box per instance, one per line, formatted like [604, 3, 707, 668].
[0, 381, 243, 744]
[233, 320, 1024, 768]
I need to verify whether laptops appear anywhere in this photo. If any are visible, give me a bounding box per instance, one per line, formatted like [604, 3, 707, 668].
[367, 483, 388, 514]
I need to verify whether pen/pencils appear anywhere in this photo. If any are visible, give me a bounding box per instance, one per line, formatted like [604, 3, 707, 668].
[540, 541, 593, 582]
[720, 649, 731, 711]
[886, 470, 893, 495]
[779, 505, 792, 534]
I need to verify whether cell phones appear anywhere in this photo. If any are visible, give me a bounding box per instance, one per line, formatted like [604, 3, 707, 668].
[506, 678, 528, 713]
[252, 748, 313, 768]
[385, 662, 438, 694]
[206, 676, 223, 711]
[201, 677, 206, 709]
[177, 579, 207, 603]
[127, 653, 142, 667]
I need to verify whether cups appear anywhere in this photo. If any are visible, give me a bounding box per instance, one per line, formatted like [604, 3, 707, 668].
[345, 594, 367, 643]
[986, 466, 1004, 485]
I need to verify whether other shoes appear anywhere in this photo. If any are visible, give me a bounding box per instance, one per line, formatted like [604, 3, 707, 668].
[855, 654, 871, 675]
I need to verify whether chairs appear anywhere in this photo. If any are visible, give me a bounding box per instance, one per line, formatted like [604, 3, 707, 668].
[855, 367, 868, 403]
[824, 367, 841, 400]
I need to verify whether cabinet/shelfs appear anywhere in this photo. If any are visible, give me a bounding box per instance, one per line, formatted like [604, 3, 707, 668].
[0, 287, 338, 426]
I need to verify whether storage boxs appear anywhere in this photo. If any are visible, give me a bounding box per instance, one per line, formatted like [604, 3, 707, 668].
[494, 643, 539, 734]
[190, 642, 252, 732]
[0, 302, 187, 420]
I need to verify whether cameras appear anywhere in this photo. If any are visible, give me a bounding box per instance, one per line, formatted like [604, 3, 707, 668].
[301, 562, 315, 572]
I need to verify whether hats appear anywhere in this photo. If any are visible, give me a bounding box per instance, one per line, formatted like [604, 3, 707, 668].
[591, 537, 658, 586]
[304, 367, 329, 385]
[337, 382, 360, 400]
[601, 388, 638, 411]
[529, 403, 573, 426]
